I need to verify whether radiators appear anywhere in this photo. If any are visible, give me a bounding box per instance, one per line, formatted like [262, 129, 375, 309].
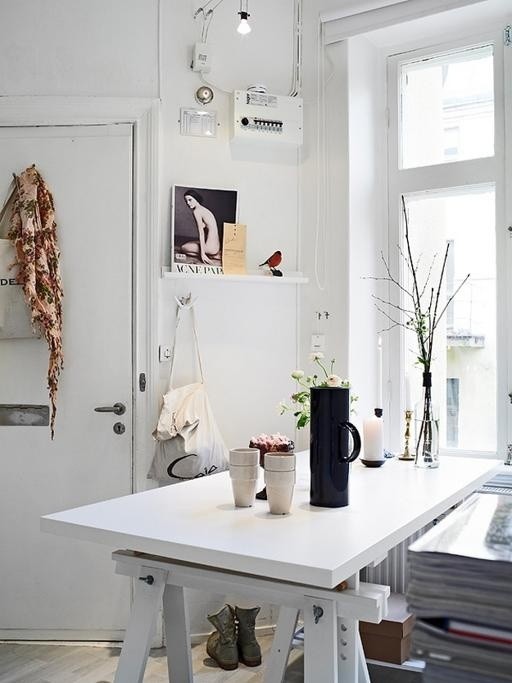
[363, 526, 423, 594]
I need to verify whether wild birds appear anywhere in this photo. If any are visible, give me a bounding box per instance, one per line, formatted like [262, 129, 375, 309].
[259, 251, 282, 272]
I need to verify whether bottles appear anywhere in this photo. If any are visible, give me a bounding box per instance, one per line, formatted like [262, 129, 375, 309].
[414, 372, 440, 468]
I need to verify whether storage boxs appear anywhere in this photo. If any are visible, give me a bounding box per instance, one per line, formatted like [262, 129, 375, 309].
[357, 592, 414, 665]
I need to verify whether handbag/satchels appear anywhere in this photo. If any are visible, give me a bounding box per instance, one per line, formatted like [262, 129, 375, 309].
[147, 383, 230, 482]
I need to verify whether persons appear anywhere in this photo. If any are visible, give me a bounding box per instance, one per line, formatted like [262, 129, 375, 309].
[178, 190, 223, 266]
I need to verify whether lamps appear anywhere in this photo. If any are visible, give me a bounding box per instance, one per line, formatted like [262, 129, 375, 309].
[195, 87, 213, 110]
[191, 0, 252, 76]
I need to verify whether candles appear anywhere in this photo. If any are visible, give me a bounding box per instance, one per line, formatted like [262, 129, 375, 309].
[364, 420, 384, 462]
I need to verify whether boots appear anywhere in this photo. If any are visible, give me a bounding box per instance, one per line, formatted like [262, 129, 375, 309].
[207, 604, 261, 670]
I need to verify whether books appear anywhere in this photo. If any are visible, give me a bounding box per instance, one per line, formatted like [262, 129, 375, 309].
[401, 488, 512, 682]
[168, 180, 240, 275]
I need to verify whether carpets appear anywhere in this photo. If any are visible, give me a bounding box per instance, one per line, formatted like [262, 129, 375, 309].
[284, 652, 423, 682]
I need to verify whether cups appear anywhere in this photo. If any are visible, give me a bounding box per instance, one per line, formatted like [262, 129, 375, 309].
[264, 451, 297, 516]
[229, 448, 261, 508]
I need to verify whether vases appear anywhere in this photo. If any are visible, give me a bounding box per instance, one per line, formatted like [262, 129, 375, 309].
[413, 385, 440, 469]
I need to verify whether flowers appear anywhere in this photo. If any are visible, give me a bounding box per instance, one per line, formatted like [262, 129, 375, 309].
[280, 351, 359, 430]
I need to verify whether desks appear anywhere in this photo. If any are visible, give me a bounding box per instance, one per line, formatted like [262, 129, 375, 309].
[41, 450, 506, 683]
